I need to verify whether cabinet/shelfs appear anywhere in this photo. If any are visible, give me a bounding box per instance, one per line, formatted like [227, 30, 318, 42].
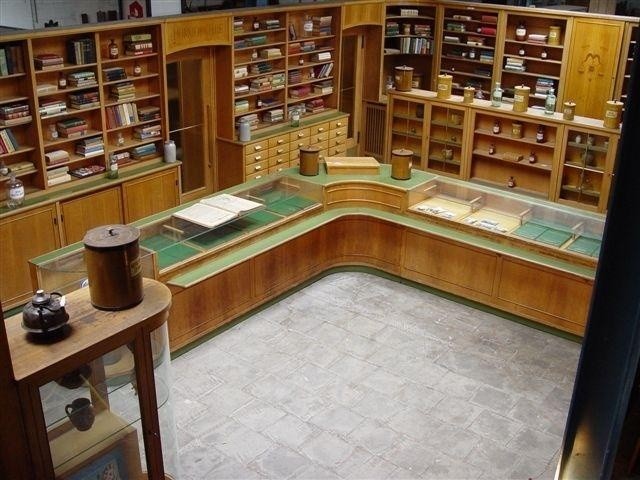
[1, 21, 169, 202]
[2, 165, 179, 308]
[231, 3, 341, 138]
[217, 116, 348, 188]
[385, 0, 639, 126]
[385, 89, 621, 214]
[2, 277, 174, 480]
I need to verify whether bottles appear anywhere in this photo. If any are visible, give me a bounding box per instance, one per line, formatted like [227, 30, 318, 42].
[251, 48, 258, 61]
[239, 120, 250, 142]
[545, 89, 557, 116]
[291, 108, 300, 128]
[134, 61, 142, 77]
[507, 176, 514, 188]
[491, 82, 502, 107]
[252, 16, 261, 32]
[298, 55, 305, 67]
[255, 95, 263, 108]
[108, 38, 118, 58]
[107, 153, 119, 180]
[164, 139, 177, 162]
[58, 72, 67, 89]
[4, 176, 25, 210]
[529, 150, 536, 164]
[536, 124, 546, 143]
[488, 143, 496, 155]
[493, 123, 502, 136]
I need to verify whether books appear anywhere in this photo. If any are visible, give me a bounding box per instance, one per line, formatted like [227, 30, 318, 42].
[383, 7, 560, 105]
[233, 11, 333, 128]
[0, 33, 164, 188]
[510, 221, 603, 262]
[323, 156, 380, 173]
[132, 189, 317, 270]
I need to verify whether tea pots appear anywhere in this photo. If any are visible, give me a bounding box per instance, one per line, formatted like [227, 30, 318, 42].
[20, 290, 69, 335]
[65, 398, 96, 431]
